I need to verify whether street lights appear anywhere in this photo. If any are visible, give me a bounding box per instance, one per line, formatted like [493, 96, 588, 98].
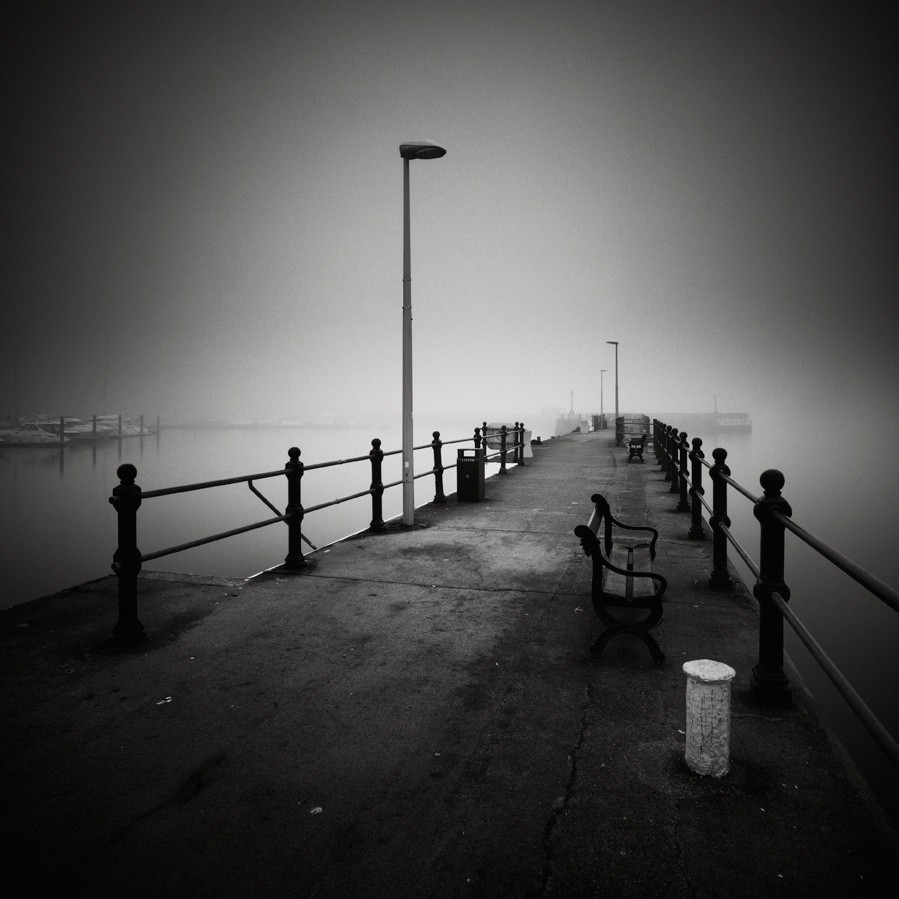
[601, 370, 608, 429]
[398, 142, 447, 523]
[604, 341, 619, 439]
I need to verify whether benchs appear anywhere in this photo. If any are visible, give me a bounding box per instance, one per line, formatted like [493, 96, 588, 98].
[628, 434, 647, 463]
[574, 494, 667, 664]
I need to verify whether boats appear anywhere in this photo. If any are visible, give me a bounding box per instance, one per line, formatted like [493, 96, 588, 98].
[555, 388, 590, 437]
[0, 414, 156, 445]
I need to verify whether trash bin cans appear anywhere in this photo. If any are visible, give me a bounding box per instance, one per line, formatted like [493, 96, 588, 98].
[456, 448, 485, 503]
